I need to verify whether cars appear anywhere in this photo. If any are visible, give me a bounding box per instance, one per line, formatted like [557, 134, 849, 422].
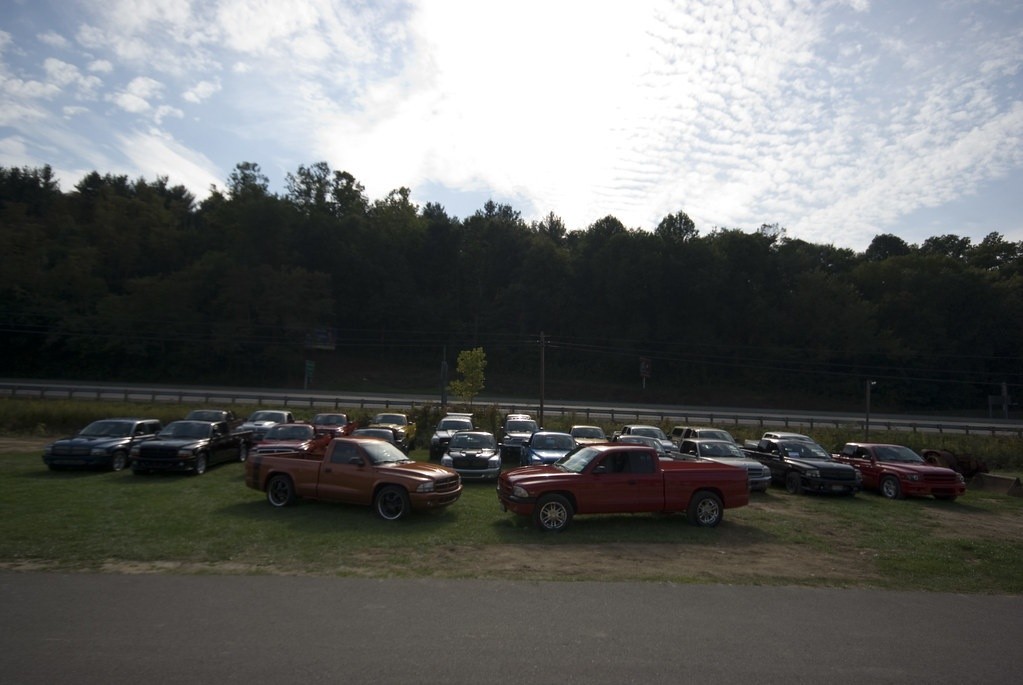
[185, 410, 247, 430]
[430, 412, 480, 459]
[441, 429, 504, 482]
[501, 414, 543, 454]
[521, 431, 577, 467]
[41, 418, 162, 472]
[569, 425, 611, 445]
[352, 427, 409, 456]
[613, 425, 678, 452]
[613, 434, 675, 461]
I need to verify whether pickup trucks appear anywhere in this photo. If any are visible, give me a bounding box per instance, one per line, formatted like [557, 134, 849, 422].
[667, 425, 826, 456]
[128, 420, 253, 476]
[739, 438, 863, 500]
[368, 413, 417, 450]
[496, 443, 749, 534]
[307, 414, 358, 439]
[236, 409, 332, 455]
[832, 443, 966, 500]
[244, 436, 464, 522]
[667, 438, 772, 493]
